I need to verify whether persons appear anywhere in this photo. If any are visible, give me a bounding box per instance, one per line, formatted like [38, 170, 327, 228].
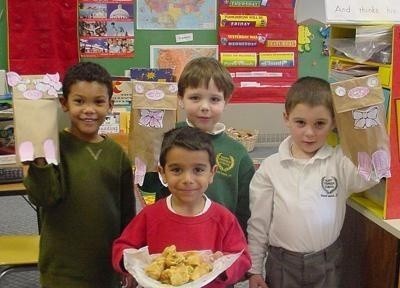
[24, 60, 141, 288]
[110, 126, 252, 287]
[243, 75, 384, 287]
[80, 14, 134, 54]
[137, 56, 255, 245]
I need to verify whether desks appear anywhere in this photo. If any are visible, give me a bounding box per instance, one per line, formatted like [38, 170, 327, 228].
[0, 165, 41, 234]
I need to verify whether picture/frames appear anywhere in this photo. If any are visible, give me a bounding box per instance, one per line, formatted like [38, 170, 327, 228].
[149, 44, 220, 79]
[135, 0, 217, 31]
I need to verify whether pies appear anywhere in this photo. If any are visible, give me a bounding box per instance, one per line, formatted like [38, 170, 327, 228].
[145, 245, 213, 286]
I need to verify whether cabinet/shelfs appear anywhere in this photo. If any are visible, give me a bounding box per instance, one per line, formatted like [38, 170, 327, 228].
[327, 23, 400, 220]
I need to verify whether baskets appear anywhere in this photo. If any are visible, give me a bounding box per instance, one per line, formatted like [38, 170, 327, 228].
[226, 130, 257, 152]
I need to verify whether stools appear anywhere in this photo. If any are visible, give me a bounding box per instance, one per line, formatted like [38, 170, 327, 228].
[0, 234, 41, 279]
